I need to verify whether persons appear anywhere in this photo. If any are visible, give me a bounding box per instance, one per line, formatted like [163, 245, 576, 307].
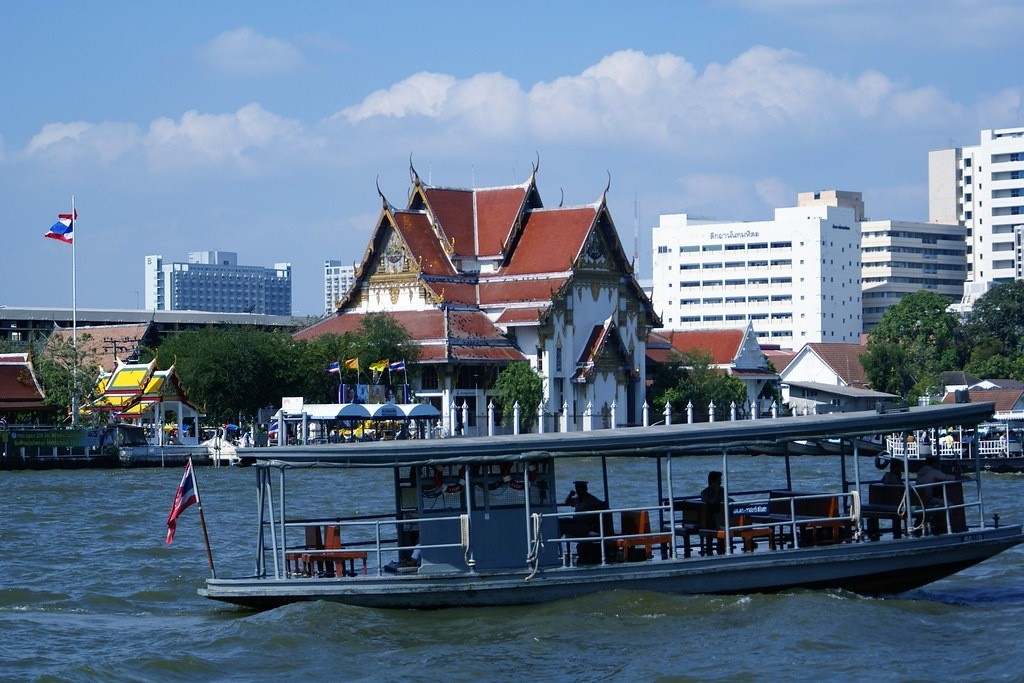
[906, 427, 1019, 457]
[883, 458, 905, 485]
[348, 387, 362, 404]
[914, 456, 971, 519]
[700, 471, 726, 556]
[558, 481, 606, 539]
[385, 388, 396, 405]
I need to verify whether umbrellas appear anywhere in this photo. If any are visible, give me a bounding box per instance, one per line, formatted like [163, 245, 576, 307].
[223, 424, 239, 430]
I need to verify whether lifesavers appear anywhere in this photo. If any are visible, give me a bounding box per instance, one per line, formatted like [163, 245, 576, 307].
[874, 451, 890, 469]
[407, 457, 540, 499]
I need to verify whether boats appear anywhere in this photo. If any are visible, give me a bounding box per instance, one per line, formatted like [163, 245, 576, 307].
[197, 391, 1024, 608]
[886, 411, 1024, 473]
[117, 442, 212, 465]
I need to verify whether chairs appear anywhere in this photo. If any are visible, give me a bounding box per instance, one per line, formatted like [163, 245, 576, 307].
[598, 509, 673, 559]
[285, 524, 368, 576]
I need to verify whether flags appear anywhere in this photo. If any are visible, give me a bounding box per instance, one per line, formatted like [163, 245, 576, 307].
[44, 208, 78, 244]
[165, 457, 201, 546]
[344, 358, 359, 368]
[388, 361, 405, 372]
[327, 362, 340, 373]
[268, 420, 279, 437]
[369, 359, 389, 372]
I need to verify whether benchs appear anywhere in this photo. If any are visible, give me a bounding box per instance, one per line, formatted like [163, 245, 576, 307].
[662, 486, 855, 556]
[860, 484, 912, 541]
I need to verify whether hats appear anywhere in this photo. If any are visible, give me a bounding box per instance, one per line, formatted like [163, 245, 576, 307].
[573, 481, 589, 487]
